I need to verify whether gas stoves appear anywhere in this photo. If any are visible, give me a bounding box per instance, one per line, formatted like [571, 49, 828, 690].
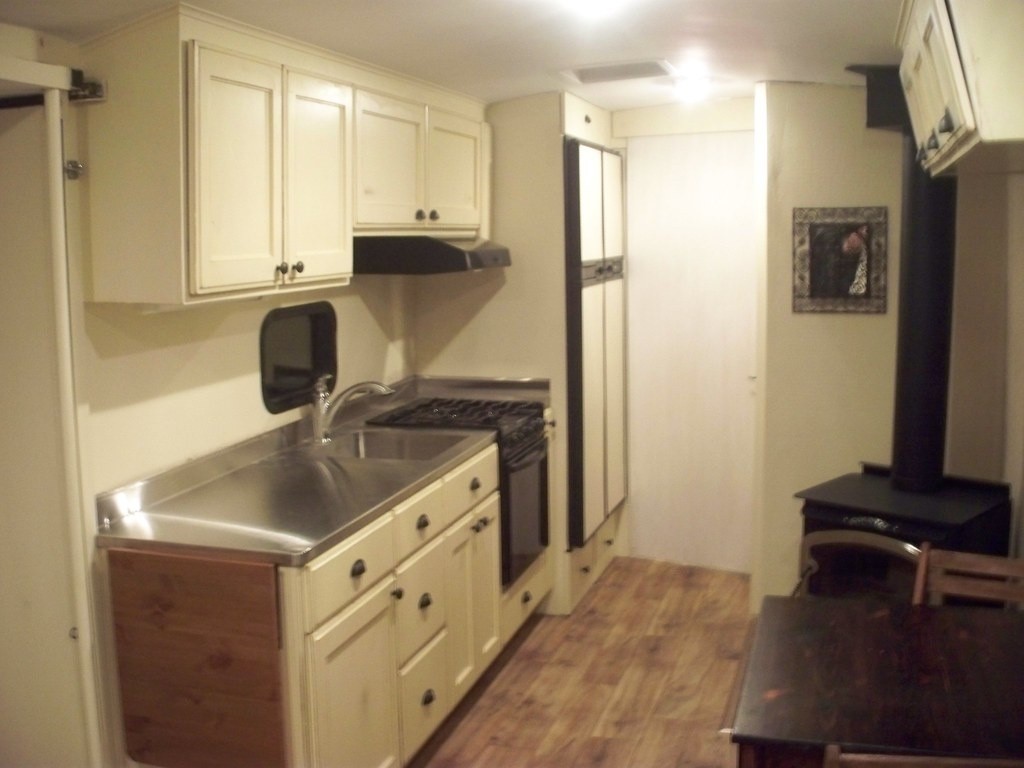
[366, 398, 545, 437]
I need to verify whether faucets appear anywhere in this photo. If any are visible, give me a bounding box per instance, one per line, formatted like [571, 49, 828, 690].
[314, 374, 397, 448]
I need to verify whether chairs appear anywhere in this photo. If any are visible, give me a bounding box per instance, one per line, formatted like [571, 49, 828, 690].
[911, 542, 1024, 610]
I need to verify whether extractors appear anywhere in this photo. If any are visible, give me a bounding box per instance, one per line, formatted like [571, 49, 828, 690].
[352, 235, 512, 276]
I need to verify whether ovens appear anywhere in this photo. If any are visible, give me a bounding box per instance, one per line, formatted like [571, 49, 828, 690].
[500, 436, 548, 585]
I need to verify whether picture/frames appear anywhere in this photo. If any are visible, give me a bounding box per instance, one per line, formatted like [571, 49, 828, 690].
[790, 205, 888, 314]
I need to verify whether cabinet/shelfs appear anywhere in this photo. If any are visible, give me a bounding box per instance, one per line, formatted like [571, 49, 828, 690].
[414, 91, 632, 614]
[890, 0, 1024, 179]
[79, 1, 493, 305]
[95, 440, 558, 768]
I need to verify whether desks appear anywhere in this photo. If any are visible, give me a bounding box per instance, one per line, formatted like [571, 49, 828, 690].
[729, 597, 1024, 768]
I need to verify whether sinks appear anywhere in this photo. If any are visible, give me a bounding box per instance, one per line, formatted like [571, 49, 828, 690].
[313, 426, 472, 468]
